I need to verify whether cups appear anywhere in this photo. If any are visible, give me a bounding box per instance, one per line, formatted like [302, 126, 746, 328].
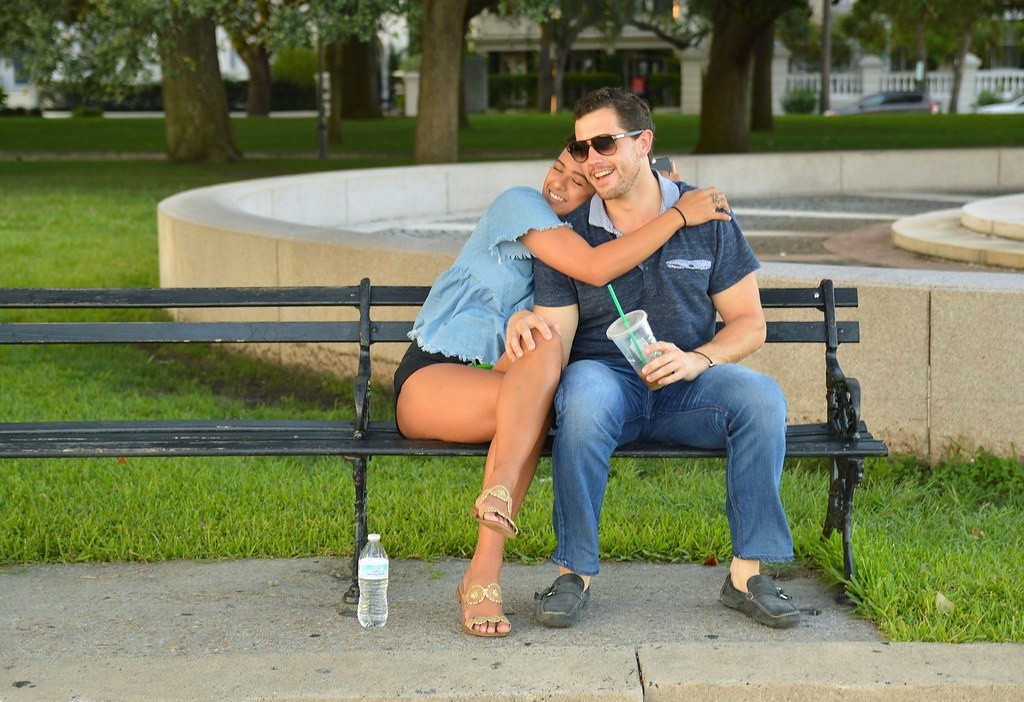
[606, 309, 672, 390]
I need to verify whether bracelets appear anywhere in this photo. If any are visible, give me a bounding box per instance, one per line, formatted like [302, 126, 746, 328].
[688, 350, 715, 367]
[669, 207, 687, 228]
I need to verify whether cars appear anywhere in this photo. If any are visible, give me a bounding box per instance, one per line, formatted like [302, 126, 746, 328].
[823, 92, 942, 118]
[975, 95, 1024, 122]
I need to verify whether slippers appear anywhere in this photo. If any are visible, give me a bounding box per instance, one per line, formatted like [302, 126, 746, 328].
[471, 485, 518, 539]
[456, 583, 511, 637]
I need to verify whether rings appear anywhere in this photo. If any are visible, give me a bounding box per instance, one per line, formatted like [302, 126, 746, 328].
[713, 192, 722, 209]
[668, 170, 672, 176]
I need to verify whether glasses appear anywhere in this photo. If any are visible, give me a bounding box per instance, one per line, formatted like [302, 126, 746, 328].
[567, 130, 644, 164]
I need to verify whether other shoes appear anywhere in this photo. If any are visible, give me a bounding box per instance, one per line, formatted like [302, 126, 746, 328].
[534, 572, 589, 627]
[719, 573, 800, 629]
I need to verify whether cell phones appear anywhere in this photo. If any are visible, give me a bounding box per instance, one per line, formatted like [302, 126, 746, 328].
[652, 157, 672, 172]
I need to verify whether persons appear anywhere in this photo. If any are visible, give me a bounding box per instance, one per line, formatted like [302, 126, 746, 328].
[392, 133, 732, 638]
[504, 87, 802, 630]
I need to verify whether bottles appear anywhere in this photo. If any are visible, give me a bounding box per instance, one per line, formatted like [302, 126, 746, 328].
[357, 533, 389, 628]
